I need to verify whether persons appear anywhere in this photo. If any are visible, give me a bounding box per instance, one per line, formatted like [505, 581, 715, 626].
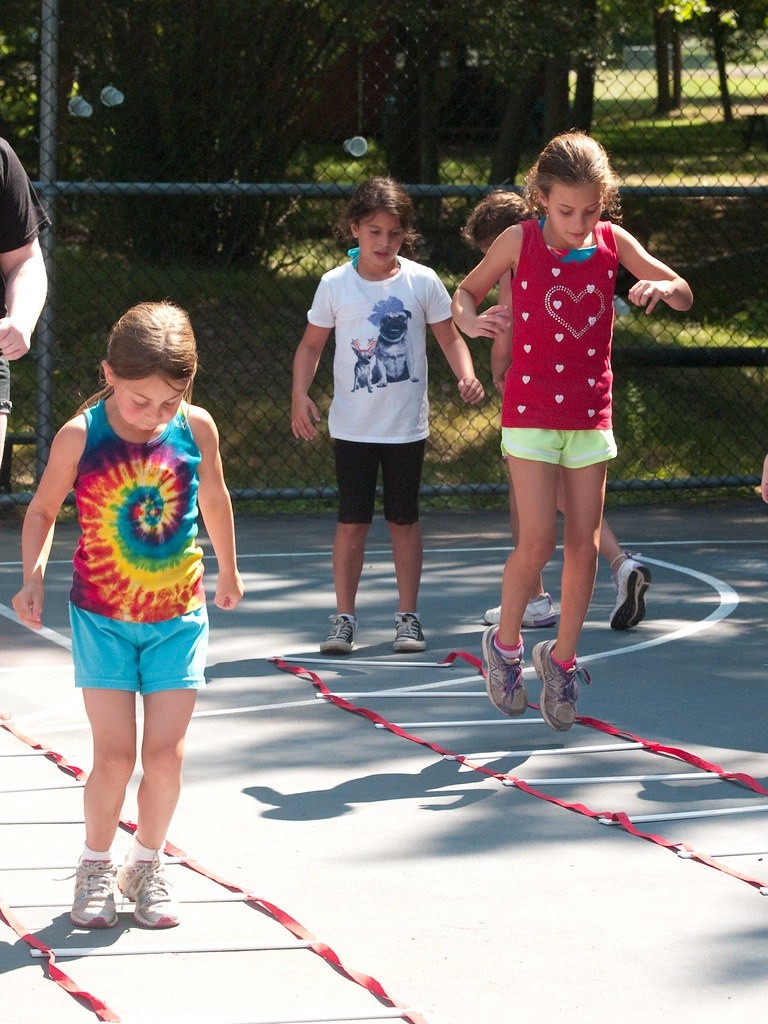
[12, 301, 245, 928]
[0, 135, 54, 467]
[291, 177, 485, 651]
[464, 190, 651, 630]
[452, 132, 692, 731]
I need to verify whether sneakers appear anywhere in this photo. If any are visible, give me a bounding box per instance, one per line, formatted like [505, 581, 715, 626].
[117, 849, 180, 929]
[610, 551, 651, 630]
[393, 611, 426, 650]
[483, 592, 557, 627]
[52, 854, 118, 928]
[482, 624, 527, 717]
[320, 615, 358, 652]
[532, 639, 591, 731]
